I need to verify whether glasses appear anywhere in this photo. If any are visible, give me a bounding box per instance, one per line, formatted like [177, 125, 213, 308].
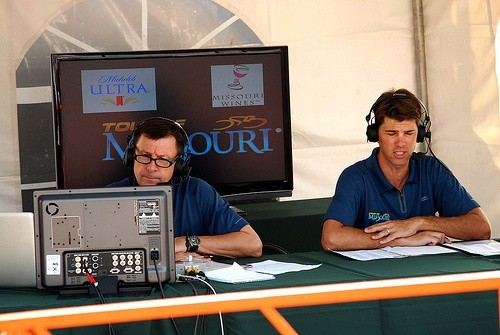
[132, 151, 179, 168]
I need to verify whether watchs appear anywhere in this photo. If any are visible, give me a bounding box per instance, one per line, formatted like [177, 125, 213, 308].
[188, 236, 201, 252]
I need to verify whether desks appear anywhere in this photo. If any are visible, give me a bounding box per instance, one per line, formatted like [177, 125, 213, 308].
[0, 248, 500, 335]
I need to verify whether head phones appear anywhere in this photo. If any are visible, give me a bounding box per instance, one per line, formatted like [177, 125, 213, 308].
[123, 116, 193, 186]
[366, 93, 431, 143]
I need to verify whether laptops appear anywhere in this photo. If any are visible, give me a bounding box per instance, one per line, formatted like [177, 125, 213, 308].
[0, 212, 37, 290]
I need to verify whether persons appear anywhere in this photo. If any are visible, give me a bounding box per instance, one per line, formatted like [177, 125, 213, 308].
[320, 88, 492, 251]
[104, 117, 263, 257]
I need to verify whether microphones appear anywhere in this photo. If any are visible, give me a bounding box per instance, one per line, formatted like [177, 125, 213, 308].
[157, 181, 182, 185]
[417, 142, 430, 159]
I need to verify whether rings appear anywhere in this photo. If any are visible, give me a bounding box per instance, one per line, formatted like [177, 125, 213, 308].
[386, 229, 390, 233]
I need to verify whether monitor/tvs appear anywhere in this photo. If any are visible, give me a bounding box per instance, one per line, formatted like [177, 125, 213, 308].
[50, 46, 293, 203]
[33, 185, 175, 299]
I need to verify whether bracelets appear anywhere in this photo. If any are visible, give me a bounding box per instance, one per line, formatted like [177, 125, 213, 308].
[185, 233, 189, 252]
